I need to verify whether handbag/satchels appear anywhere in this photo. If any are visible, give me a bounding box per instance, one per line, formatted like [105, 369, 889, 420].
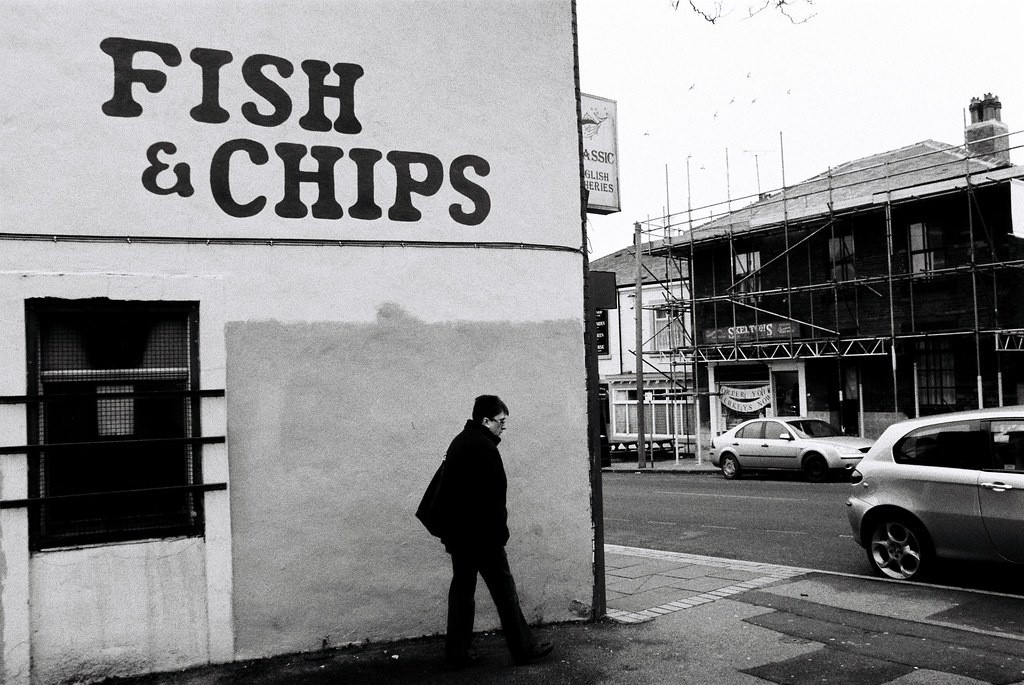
[414, 453, 445, 539]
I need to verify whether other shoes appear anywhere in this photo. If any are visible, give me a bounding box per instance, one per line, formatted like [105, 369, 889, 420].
[515, 642, 554, 666]
[451, 648, 481, 663]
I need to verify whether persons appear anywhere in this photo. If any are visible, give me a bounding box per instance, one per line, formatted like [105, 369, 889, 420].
[440, 394, 555, 670]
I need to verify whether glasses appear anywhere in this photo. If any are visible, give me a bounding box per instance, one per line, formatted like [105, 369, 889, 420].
[491, 418, 505, 426]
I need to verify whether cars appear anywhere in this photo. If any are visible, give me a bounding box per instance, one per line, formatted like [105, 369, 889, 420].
[845, 404, 1024, 582]
[709, 416, 876, 483]
